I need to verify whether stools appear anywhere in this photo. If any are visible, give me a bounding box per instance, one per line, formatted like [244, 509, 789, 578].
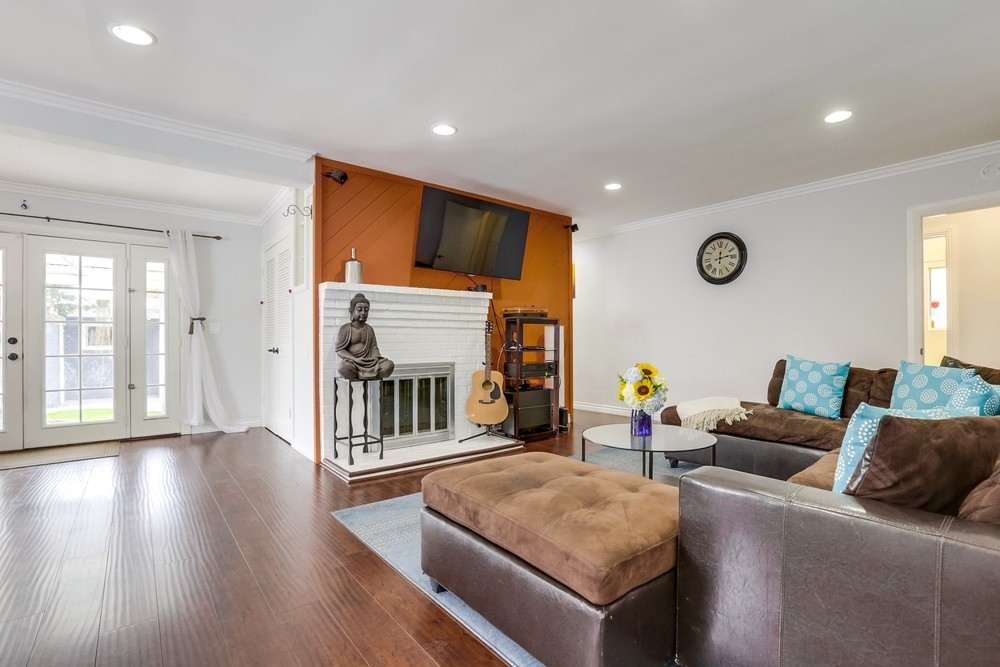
[420, 454, 677, 667]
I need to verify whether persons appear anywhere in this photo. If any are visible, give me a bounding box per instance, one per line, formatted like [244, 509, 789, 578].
[334, 293, 394, 380]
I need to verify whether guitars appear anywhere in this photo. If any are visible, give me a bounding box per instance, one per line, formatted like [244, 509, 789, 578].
[465, 319, 510, 428]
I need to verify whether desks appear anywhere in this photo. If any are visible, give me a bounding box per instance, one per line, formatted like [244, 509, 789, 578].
[333, 376, 384, 465]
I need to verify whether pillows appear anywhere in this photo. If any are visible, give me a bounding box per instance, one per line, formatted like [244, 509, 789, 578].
[832, 402, 980, 494]
[778, 354, 853, 420]
[889, 360, 976, 409]
[945, 375, 1000, 416]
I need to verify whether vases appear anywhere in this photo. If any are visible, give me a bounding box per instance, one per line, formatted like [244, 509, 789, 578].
[631, 408, 651, 437]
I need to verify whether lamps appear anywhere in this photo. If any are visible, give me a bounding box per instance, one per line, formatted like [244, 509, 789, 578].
[563, 223, 579, 233]
[322, 169, 349, 185]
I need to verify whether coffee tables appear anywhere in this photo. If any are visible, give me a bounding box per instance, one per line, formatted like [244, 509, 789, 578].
[581, 424, 719, 480]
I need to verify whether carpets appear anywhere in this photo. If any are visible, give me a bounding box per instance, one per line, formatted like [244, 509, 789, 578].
[330, 447, 701, 667]
[0, 441, 121, 471]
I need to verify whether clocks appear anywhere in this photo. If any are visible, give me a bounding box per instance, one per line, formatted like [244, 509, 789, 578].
[696, 231, 747, 285]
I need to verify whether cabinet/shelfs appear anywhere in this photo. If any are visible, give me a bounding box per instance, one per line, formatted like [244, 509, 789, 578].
[503, 317, 560, 442]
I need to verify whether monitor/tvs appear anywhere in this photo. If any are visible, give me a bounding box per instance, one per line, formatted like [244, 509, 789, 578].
[414, 185, 530, 281]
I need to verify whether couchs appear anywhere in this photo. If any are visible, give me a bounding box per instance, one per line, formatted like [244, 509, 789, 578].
[661, 358, 1000, 667]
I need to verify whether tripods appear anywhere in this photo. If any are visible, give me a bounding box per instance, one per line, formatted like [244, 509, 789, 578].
[459, 362, 519, 444]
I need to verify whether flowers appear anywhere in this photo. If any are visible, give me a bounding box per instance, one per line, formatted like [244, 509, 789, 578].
[616, 362, 668, 416]
